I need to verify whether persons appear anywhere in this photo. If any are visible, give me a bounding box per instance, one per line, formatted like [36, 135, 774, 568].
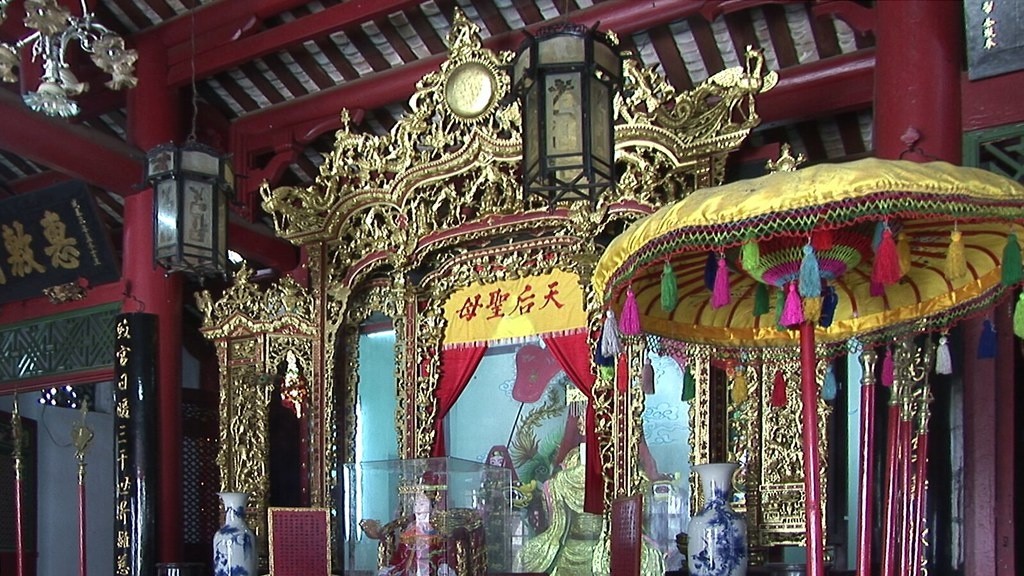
[377, 472, 459, 575]
[472, 442, 522, 532]
[515, 384, 666, 575]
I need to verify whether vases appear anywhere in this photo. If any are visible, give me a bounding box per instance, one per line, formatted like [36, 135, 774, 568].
[214, 492, 258, 575]
[688, 463, 749, 576]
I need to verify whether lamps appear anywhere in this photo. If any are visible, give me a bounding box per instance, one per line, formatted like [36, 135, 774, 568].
[0, 0, 139, 118]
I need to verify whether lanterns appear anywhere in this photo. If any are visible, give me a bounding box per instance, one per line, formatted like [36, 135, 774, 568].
[496, 20, 622, 212]
[142, 141, 247, 287]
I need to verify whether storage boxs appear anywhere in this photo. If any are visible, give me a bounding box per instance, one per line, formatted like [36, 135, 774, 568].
[342, 456, 513, 575]
